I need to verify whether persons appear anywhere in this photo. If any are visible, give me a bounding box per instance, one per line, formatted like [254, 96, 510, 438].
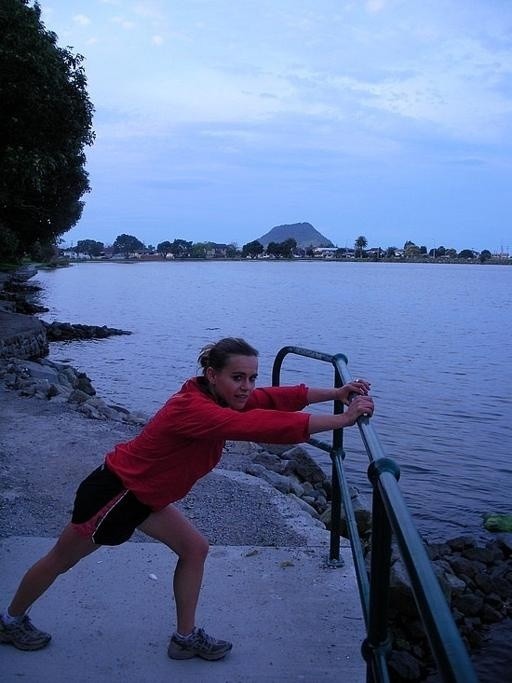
[1, 333, 377, 659]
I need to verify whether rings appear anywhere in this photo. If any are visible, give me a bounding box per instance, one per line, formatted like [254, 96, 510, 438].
[355, 379, 358, 383]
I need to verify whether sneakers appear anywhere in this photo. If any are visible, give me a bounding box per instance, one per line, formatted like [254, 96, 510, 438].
[167, 626, 232, 661]
[0, 607, 51, 650]
